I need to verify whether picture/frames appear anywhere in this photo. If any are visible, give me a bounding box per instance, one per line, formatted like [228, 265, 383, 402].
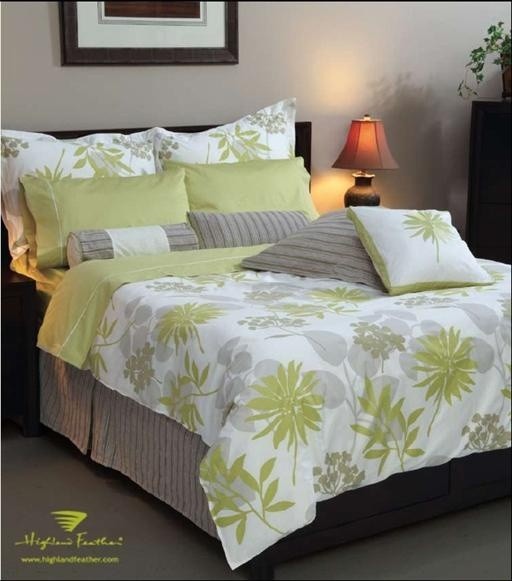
[58, 1, 241, 69]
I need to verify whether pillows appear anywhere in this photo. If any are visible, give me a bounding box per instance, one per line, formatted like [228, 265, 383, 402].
[1, 98, 494, 296]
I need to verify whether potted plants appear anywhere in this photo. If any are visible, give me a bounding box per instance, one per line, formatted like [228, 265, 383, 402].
[455, 21, 512, 99]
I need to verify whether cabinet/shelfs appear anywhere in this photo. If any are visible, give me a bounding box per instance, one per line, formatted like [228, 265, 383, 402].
[463, 99, 510, 265]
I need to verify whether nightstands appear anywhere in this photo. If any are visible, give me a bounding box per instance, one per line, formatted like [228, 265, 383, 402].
[3, 268, 45, 437]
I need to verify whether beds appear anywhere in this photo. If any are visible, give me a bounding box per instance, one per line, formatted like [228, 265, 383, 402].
[2, 122, 512, 581]
